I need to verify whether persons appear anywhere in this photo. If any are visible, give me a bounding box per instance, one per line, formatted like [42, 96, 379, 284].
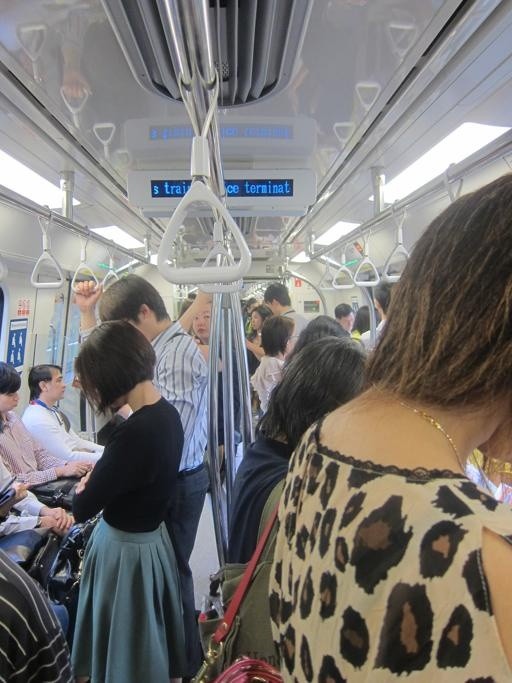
[60, 12, 93, 99]
[1, 170, 511, 683]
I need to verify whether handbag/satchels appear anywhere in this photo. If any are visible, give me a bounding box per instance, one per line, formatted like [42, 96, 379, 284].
[0, 529, 59, 582]
[50, 524, 91, 605]
[34, 473, 79, 513]
[197, 561, 283, 682]
